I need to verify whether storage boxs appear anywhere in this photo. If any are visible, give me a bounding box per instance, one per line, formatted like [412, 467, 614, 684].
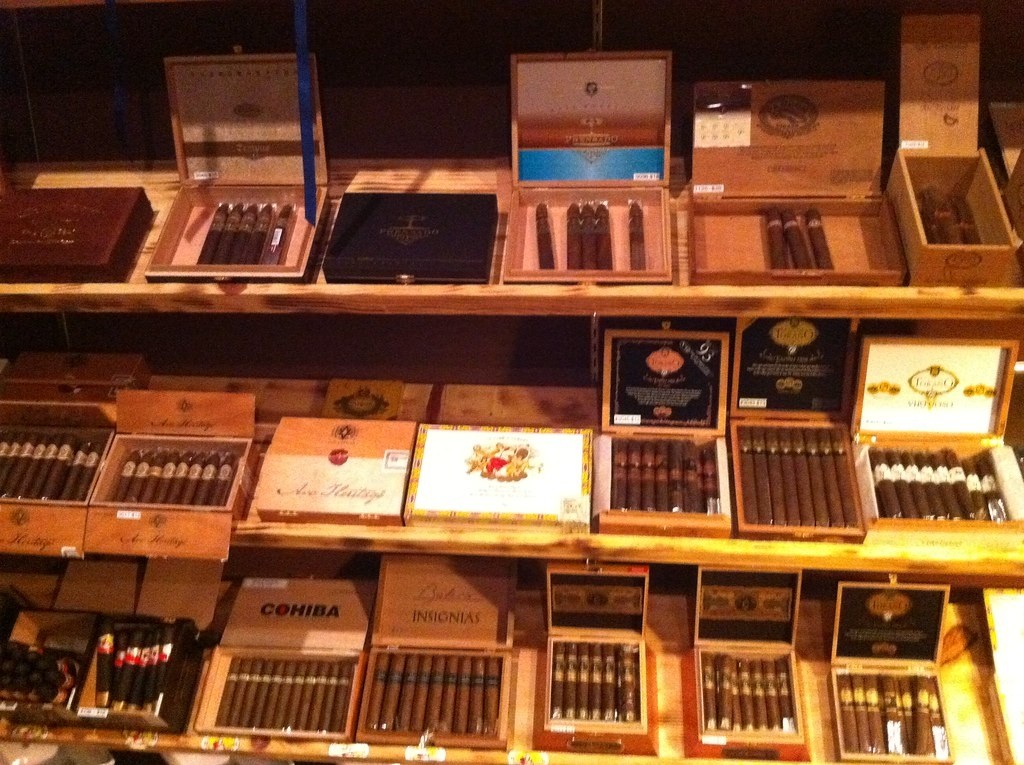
[144, 45, 331, 284]
[543, 558, 649, 751]
[257, 416, 417, 527]
[887, 13, 1016, 286]
[0, 187, 153, 284]
[322, 193, 499, 285]
[0, 353, 256, 729]
[990, 101, 1024, 247]
[355, 555, 517, 752]
[404, 423, 593, 533]
[599, 328, 732, 538]
[729, 316, 867, 545]
[502, 50, 673, 286]
[688, 79, 908, 287]
[688, 563, 805, 761]
[194, 574, 378, 741]
[850, 338, 1024, 534]
[827, 582, 955, 765]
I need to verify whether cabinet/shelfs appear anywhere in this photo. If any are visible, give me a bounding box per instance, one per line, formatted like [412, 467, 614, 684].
[0, 160, 1024, 765]
